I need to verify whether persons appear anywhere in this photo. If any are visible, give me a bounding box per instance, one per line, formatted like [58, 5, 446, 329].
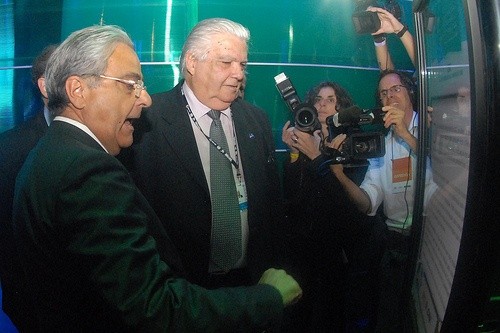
[282, 82, 369, 333]
[367, 7, 415, 72]
[123, 18, 276, 290]
[12, 25, 302, 333]
[329, 69, 419, 333]
[0, 45, 56, 316]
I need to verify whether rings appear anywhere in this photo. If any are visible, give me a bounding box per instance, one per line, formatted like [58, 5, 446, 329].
[295, 138, 298, 141]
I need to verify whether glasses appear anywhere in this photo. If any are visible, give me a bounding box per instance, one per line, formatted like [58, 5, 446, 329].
[100, 74, 147, 98]
[377, 85, 408, 100]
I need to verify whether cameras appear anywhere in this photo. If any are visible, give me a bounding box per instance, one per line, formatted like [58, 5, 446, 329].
[274, 71, 323, 135]
[351, 11, 381, 34]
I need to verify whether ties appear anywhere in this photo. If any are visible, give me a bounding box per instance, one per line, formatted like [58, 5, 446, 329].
[207, 109, 242, 272]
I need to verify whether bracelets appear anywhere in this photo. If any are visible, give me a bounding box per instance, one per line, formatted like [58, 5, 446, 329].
[374, 37, 387, 46]
[290, 152, 299, 159]
[397, 25, 408, 37]
